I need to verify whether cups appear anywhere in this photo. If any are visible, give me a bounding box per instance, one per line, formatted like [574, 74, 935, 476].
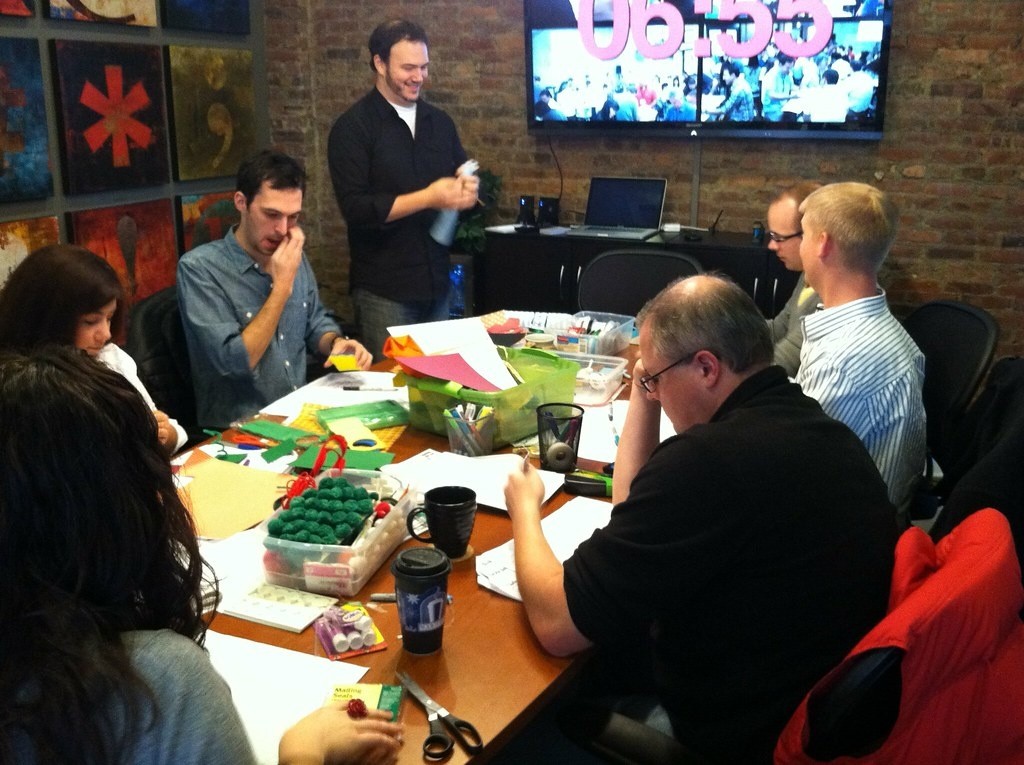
[390, 547, 454, 653]
[406, 485, 478, 559]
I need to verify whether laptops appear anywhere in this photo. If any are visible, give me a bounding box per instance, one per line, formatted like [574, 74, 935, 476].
[567, 177, 667, 240]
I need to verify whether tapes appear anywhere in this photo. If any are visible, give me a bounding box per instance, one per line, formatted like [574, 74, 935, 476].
[547, 443, 575, 469]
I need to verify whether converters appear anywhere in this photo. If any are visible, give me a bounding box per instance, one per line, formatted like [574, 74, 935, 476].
[663, 223, 681, 233]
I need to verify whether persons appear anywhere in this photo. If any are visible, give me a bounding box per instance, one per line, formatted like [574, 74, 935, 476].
[175, 150, 373, 446]
[0, 244, 188, 458]
[764, 181, 822, 379]
[328, 19, 480, 363]
[505, 270, 903, 765]
[0, 345, 403, 765]
[535, 35, 881, 123]
[787, 181, 926, 533]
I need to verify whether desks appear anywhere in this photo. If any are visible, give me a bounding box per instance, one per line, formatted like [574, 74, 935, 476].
[167, 308, 690, 765]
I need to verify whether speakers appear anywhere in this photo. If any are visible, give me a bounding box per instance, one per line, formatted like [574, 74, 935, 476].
[516, 195, 536, 225]
[536, 197, 559, 228]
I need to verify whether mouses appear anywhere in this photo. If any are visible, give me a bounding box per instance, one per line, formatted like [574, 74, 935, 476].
[685, 230, 703, 241]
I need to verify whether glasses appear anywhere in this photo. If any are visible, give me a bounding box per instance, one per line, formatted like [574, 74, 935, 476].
[640, 347, 723, 394]
[767, 230, 804, 242]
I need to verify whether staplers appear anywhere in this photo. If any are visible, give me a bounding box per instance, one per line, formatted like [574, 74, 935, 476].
[565, 468, 613, 497]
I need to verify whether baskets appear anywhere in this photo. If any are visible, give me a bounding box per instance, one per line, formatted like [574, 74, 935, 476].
[536, 402, 584, 473]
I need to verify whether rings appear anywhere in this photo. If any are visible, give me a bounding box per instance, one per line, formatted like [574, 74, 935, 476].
[347, 699, 368, 718]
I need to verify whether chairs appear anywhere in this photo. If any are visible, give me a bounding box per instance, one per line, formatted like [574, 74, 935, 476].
[130, 284, 214, 458]
[576, 250, 702, 322]
[771, 301, 1024, 765]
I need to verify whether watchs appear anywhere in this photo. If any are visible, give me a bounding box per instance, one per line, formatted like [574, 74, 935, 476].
[330, 334, 349, 352]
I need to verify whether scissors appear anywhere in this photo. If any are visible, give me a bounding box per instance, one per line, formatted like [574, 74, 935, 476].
[232, 435, 272, 449]
[394, 670, 484, 758]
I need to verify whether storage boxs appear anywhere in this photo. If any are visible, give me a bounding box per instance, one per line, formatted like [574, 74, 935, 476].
[401, 311, 636, 446]
[260, 466, 409, 597]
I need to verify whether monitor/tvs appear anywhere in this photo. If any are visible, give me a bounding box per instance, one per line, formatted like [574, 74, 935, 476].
[524, 0, 894, 141]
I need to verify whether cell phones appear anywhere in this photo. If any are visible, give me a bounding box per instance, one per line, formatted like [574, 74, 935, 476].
[603, 461, 615, 475]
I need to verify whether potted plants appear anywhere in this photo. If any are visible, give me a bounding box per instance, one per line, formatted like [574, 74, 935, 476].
[438, 171, 503, 319]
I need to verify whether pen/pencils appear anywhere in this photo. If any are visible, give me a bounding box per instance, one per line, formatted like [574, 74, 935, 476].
[371, 593, 452, 603]
[443, 402, 496, 457]
[609, 401, 613, 421]
[545, 411, 560, 439]
[219, 441, 261, 450]
[608, 414, 619, 446]
[542, 419, 580, 466]
[243, 460, 250, 466]
[343, 386, 398, 391]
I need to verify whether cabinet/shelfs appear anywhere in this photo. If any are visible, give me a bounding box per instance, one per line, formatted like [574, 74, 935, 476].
[479, 224, 799, 322]
